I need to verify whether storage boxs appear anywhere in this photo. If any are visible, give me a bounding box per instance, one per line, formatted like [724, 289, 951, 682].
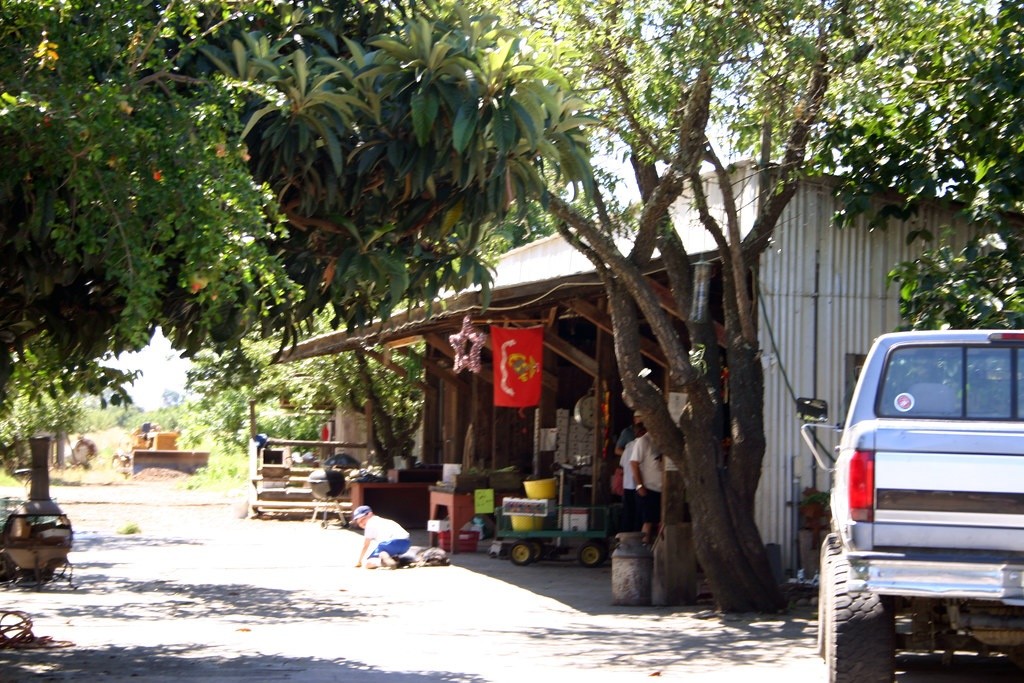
[562, 505, 591, 532]
[502, 497, 559, 518]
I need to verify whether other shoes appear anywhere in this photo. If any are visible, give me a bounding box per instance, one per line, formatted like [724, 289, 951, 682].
[379, 551, 395, 566]
[392, 556, 400, 564]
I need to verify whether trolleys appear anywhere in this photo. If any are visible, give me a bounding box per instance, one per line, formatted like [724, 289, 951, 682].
[494, 506, 610, 567]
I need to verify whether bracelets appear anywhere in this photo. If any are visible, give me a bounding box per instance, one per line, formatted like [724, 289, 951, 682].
[636, 484, 642, 491]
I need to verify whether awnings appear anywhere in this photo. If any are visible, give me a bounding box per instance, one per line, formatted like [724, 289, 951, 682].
[270, 237, 740, 361]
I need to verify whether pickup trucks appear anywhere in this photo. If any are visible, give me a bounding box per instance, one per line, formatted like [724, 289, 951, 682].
[796, 328, 1024, 683]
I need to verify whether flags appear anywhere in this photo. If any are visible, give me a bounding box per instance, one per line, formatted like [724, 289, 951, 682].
[490, 323, 544, 407]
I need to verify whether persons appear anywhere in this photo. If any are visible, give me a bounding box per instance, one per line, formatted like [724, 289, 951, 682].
[608, 411, 664, 547]
[349, 505, 411, 568]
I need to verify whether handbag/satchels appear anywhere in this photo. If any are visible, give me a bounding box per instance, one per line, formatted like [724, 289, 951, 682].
[612, 468, 625, 496]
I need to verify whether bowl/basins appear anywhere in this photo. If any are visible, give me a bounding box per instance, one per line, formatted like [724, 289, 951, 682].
[522, 477, 557, 500]
[511, 515, 544, 532]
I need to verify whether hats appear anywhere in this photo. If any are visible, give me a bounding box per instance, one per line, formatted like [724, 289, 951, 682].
[349, 506, 371, 524]
[634, 411, 640, 416]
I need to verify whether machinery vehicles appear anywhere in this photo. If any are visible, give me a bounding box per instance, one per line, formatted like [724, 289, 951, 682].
[113, 423, 210, 476]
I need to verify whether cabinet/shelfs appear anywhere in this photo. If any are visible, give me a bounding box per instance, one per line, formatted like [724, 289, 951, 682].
[349, 482, 435, 530]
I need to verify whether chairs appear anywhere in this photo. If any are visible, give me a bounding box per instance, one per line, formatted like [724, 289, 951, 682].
[903, 383, 955, 414]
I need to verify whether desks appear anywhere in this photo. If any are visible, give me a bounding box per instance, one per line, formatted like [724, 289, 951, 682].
[427, 486, 481, 553]
